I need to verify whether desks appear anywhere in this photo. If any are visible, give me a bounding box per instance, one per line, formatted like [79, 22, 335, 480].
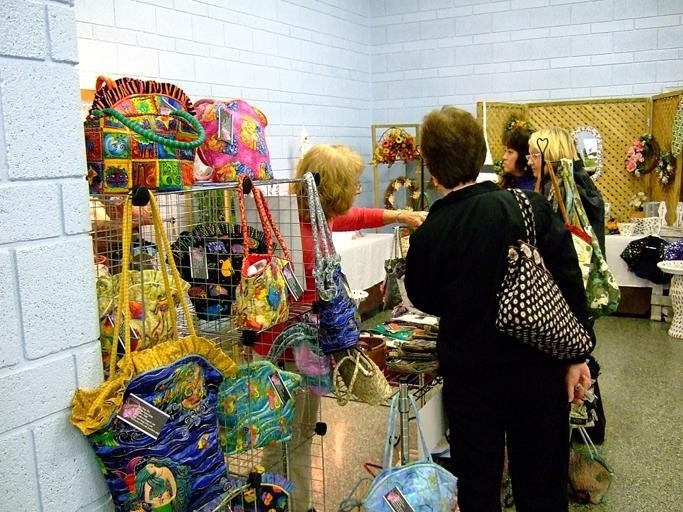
[656, 258, 683, 341]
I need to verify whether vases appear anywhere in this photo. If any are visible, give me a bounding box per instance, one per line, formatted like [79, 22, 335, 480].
[630, 211, 644, 221]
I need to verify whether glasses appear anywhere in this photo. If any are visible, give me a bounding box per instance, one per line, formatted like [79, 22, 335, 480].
[525, 153, 543, 160]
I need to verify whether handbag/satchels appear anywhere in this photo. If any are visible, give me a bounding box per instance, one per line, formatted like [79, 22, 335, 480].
[333, 349, 392, 408]
[535, 159, 621, 318]
[496, 188, 592, 361]
[569, 424, 614, 504]
[303, 173, 362, 349]
[71, 75, 333, 512]
[381, 226, 408, 310]
[359, 392, 458, 511]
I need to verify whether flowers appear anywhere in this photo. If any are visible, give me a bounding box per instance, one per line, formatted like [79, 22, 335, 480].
[629, 191, 647, 210]
[622, 131, 676, 186]
[370, 128, 419, 167]
[380, 175, 418, 215]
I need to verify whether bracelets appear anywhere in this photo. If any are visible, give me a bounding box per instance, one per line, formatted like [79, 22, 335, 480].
[393, 207, 403, 225]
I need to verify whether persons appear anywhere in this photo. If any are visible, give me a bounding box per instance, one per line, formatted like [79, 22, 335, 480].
[403, 104, 596, 512]
[495, 125, 540, 192]
[288, 143, 429, 293]
[526, 126, 607, 445]
